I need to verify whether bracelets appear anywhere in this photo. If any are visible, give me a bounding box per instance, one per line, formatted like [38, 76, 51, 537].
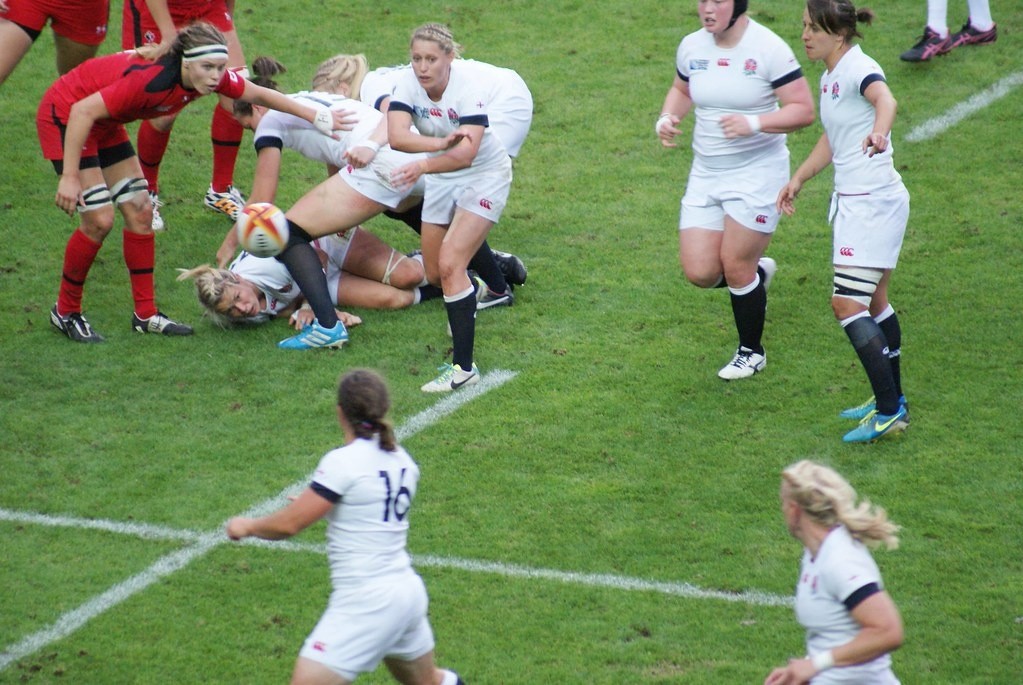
[811, 651, 834, 671]
[749, 115, 761, 133]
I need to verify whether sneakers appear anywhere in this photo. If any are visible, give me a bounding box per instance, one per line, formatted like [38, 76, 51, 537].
[949, 16, 997, 47]
[718, 345, 767, 382]
[476, 284, 514, 310]
[840, 393, 909, 420]
[50, 301, 107, 344]
[489, 248, 527, 286]
[447, 278, 488, 338]
[842, 404, 910, 444]
[276, 318, 349, 351]
[757, 257, 777, 313]
[132, 309, 193, 336]
[421, 362, 480, 393]
[901, 26, 952, 62]
[203, 182, 246, 222]
[147, 190, 165, 232]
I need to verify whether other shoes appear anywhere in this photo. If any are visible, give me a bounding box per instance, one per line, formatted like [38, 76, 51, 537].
[405, 248, 424, 259]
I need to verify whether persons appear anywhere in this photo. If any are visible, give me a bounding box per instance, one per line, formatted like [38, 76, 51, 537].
[0, 0, 111, 86]
[777, 0, 911, 442]
[655, 0, 815, 380]
[122, 0, 249, 229]
[386, 23, 513, 391]
[764, 461, 903, 685]
[37, 23, 360, 343]
[310, 56, 533, 310]
[899, 0, 998, 62]
[227, 370, 465, 685]
[216, 57, 514, 350]
[178, 225, 528, 330]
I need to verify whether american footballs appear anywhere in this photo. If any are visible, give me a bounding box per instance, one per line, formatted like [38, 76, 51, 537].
[236, 202, 290, 259]
